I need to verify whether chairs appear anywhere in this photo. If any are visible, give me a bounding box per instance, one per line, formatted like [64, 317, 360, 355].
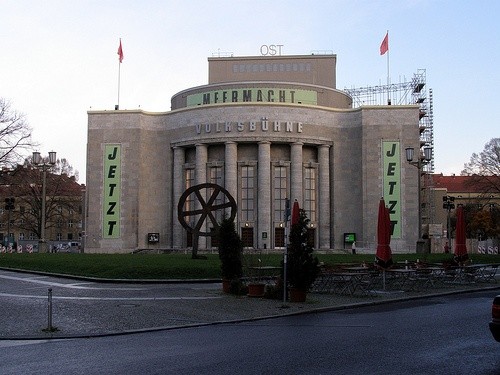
[317, 261, 500, 295]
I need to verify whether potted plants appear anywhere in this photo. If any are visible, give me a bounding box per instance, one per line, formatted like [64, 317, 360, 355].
[219, 257, 245, 293]
[278, 208, 315, 303]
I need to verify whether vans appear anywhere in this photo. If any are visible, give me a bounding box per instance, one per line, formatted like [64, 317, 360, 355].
[34, 242, 81, 253]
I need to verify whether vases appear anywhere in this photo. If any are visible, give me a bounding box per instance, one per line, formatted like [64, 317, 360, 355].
[249, 283, 265, 294]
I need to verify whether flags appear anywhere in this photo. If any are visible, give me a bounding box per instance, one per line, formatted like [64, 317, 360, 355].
[379, 31, 389, 56]
[116, 39, 125, 64]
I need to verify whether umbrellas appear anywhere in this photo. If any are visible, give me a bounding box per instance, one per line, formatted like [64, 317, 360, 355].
[372, 197, 394, 291]
[454, 204, 469, 273]
[292, 199, 300, 225]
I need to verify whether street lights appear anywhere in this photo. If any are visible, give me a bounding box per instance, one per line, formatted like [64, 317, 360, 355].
[32, 149, 57, 253]
[405, 145, 433, 253]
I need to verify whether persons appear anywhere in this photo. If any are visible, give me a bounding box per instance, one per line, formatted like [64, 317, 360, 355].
[65, 243, 70, 252]
[51, 245, 56, 254]
[444, 241, 450, 253]
[351, 241, 359, 254]
